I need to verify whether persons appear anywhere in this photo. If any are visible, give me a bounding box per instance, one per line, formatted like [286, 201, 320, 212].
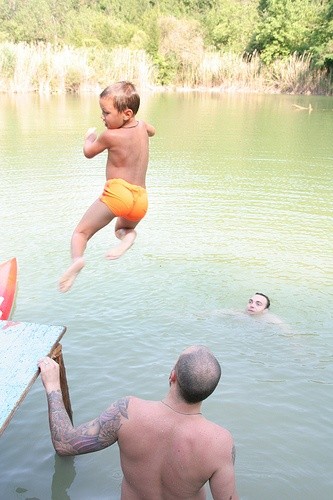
[33, 345, 239, 500]
[247, 291, 270, 316]
[55, 81, 155, 293]
